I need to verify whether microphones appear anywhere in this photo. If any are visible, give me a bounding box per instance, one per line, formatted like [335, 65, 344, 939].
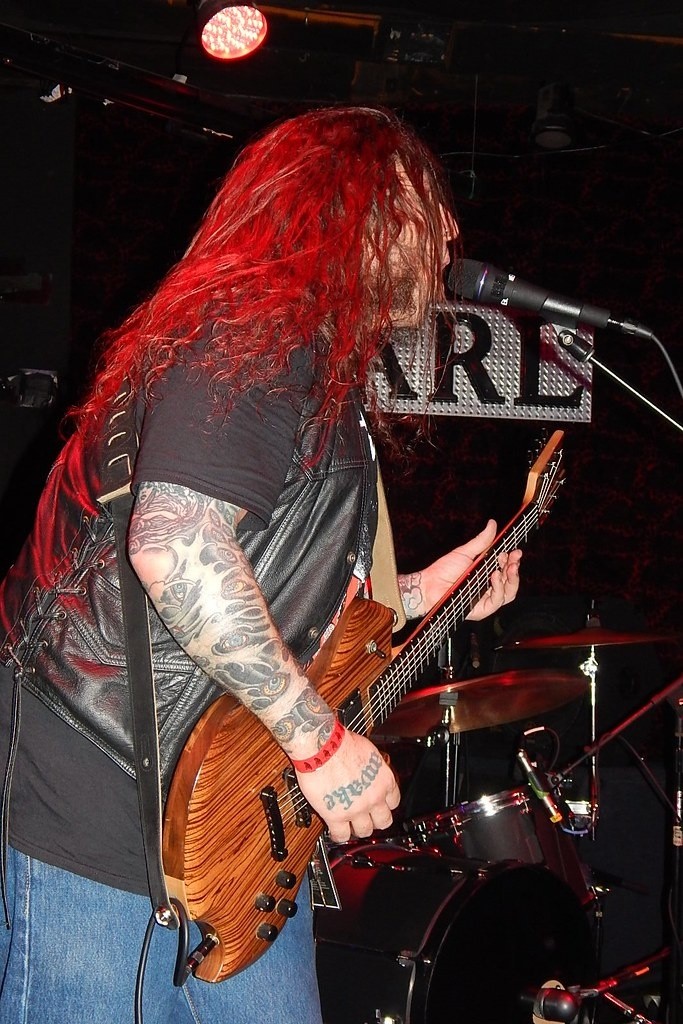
[516, 747, 563, 824]
[448, 259, 653, 342]
[518, 984, 578, 1021]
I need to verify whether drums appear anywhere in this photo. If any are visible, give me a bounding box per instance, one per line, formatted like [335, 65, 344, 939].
[313, 840, 595, 1024]
[390, 779, 605, 912]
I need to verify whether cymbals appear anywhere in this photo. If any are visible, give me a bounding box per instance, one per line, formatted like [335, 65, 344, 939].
[370, 667, 591, 741]
[491, 627, 682, 652]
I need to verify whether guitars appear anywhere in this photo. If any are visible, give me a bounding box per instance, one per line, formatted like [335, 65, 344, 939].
[162, 424, 570, 987]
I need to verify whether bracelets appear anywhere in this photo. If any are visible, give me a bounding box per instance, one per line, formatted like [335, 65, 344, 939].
[290, 716, 347, 773]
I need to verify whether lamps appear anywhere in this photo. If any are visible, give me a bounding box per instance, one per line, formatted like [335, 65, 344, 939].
[193, 0, 269, 59]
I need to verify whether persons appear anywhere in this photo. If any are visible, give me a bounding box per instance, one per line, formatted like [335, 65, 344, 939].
[3, 103, 523, 1023]
